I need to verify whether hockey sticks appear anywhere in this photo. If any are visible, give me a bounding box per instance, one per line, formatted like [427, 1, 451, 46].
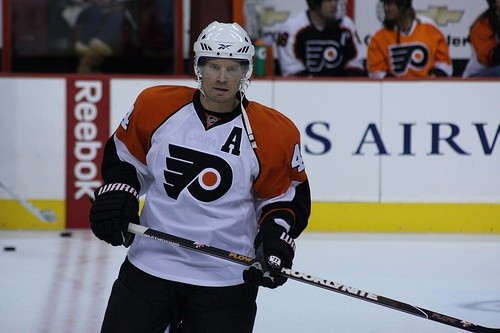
[0, 179, 59, 225]
[121, 216, 500, 333]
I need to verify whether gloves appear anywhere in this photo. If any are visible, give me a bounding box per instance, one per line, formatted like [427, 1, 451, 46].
[243, 218, 295, 289]
[89, 181, 140, 247]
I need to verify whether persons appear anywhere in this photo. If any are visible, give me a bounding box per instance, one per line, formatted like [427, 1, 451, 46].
[276, 0, 500, 80]
[88, 19, 311, 333]
[47, 0, 133, 75]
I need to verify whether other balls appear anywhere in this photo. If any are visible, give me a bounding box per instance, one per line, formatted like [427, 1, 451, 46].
[59, 232, 72, 237]
[4, 246, 16, 251]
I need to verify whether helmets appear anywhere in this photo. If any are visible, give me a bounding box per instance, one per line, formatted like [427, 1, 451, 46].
[380, 0, 411, 12]
[195, 22, 255, 80]
[306, 0, 322, 10]
